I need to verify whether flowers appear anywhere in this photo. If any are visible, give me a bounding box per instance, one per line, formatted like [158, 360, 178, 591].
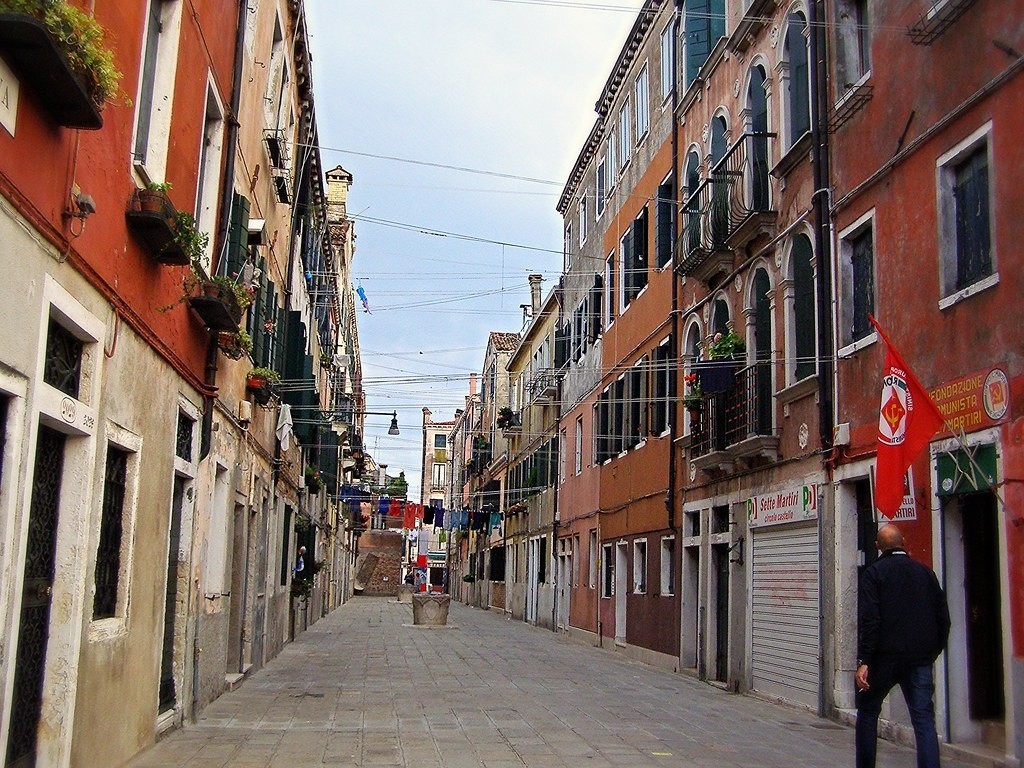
[675, 374, 704, 411]
[305, 462, 323, 489]
[211, 272, 255, 310]
[704, 332, 759, 358]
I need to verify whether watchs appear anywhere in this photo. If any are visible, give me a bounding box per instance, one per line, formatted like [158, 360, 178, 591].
[857, 659, 871, 668]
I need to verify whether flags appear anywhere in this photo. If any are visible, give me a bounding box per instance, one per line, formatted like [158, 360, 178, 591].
[866, 311, 947, 521]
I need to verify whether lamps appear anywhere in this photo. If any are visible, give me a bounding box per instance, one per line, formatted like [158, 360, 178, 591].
[312, 409, 400, 435]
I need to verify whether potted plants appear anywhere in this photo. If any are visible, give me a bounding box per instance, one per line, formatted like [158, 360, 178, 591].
[294, 515, 310, 533]
[0, 0, 135, 112]
[216, 324, 253, 361]
[138, 181, 177, 221]
[154, 209, 210, 313]
[247, 366, 283, 406]
[291, 577, 312, 611]
[464, 405, 522, 472]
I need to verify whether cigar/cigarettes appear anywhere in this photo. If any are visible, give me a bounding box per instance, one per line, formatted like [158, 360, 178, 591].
[859, 688, 863, 692]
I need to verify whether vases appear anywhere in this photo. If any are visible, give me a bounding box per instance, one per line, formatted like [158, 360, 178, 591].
[203, 283, 242, 326]
[689, 409, 701, 422]
[305, 475, 322, 494]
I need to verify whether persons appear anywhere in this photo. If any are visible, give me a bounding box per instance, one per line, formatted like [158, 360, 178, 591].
[852, 524, 952, 768]
[404, 569, 415, 586]
[416, 573, 422, 593]
